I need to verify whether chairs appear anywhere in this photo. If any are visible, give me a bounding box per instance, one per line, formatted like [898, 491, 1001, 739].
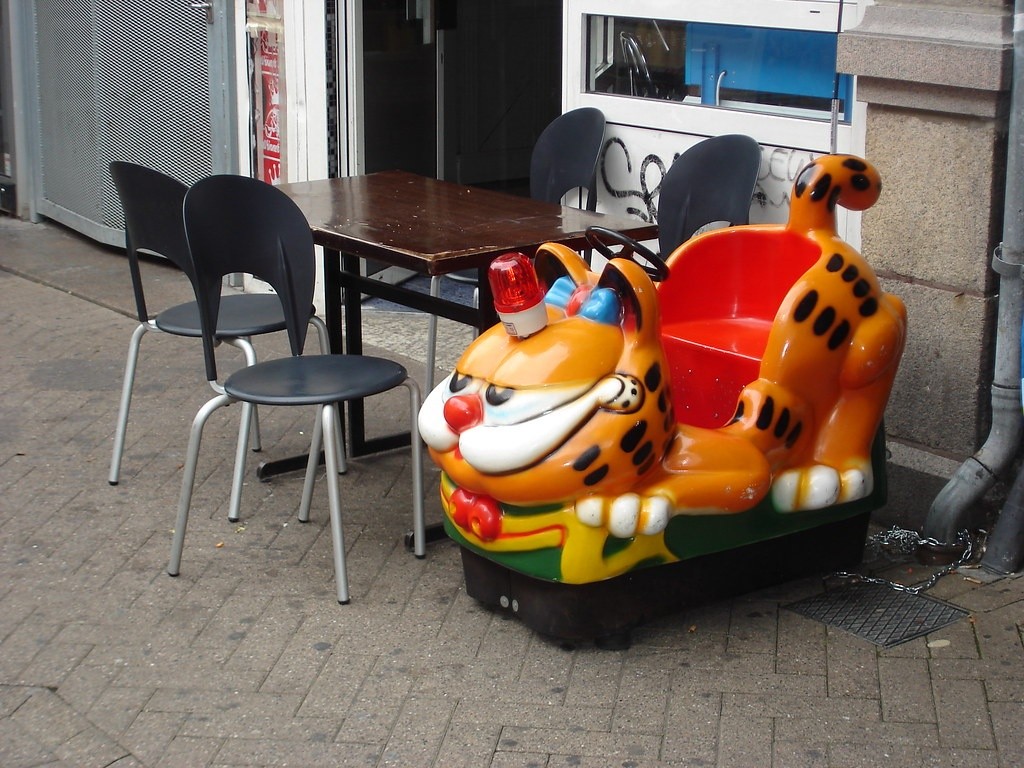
[663, 231, 821, 388]
[107, 159, 348, 521]
[166, 174, 422, 605]
[426, 108, 608, 397]
[657, 133, 761, 261]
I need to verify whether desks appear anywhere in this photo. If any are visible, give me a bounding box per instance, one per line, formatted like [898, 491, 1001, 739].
[256, 171, 659, 545]
